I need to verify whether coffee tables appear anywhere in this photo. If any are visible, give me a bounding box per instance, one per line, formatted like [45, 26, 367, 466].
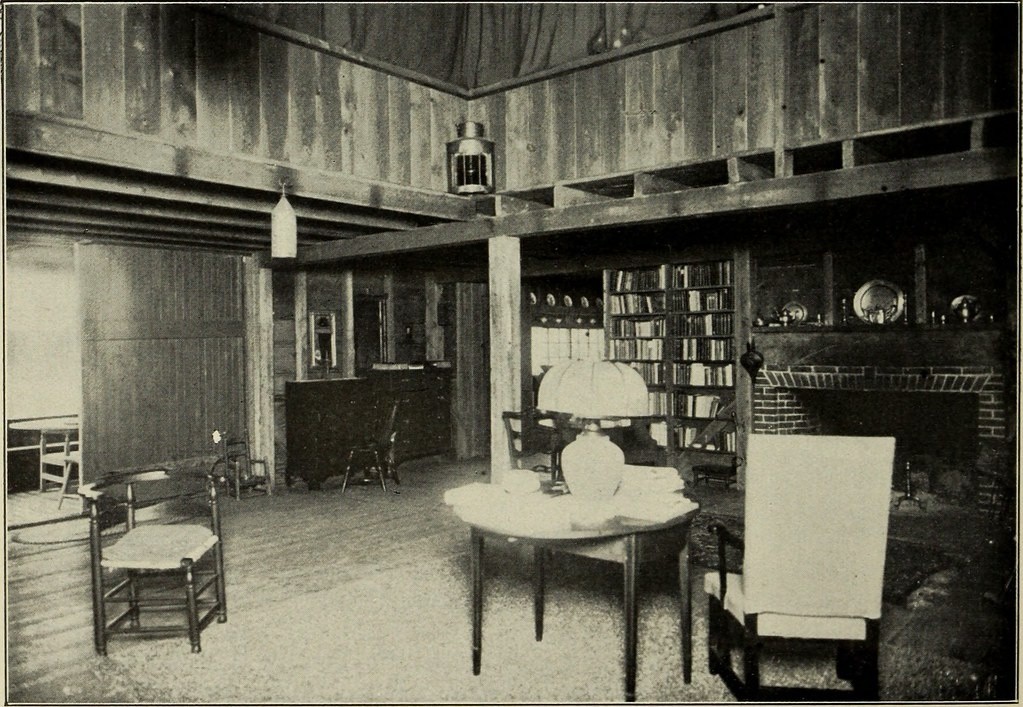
[446, 480, 704, 700]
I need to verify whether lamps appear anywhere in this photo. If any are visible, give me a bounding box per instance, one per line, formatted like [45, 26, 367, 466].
[270, 183, 297, 259]
[536, 360, 652, 497]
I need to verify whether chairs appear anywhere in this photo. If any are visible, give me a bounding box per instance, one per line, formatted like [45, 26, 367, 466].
[341, 399, 411, 494]
[75, 465, 230, 657]
[502, 411, 565, 472]
[702, 435, 898, 699]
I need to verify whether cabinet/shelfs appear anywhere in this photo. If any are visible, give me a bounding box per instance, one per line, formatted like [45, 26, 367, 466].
[600, 261, 736, 482]
[373, 367, 454, 484]
[286, 377, 381, 490]
